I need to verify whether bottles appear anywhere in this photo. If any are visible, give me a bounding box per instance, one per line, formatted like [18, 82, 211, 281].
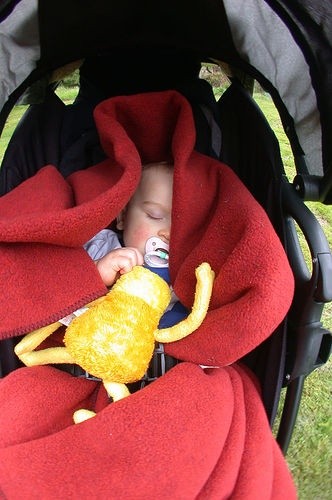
[69, 261, 175, 387]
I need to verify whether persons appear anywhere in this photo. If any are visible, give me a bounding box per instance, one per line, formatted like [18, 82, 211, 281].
[1, 151, 257, 499]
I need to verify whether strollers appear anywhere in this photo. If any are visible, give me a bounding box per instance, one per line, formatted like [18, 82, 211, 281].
[0, 0, 332, 459]
